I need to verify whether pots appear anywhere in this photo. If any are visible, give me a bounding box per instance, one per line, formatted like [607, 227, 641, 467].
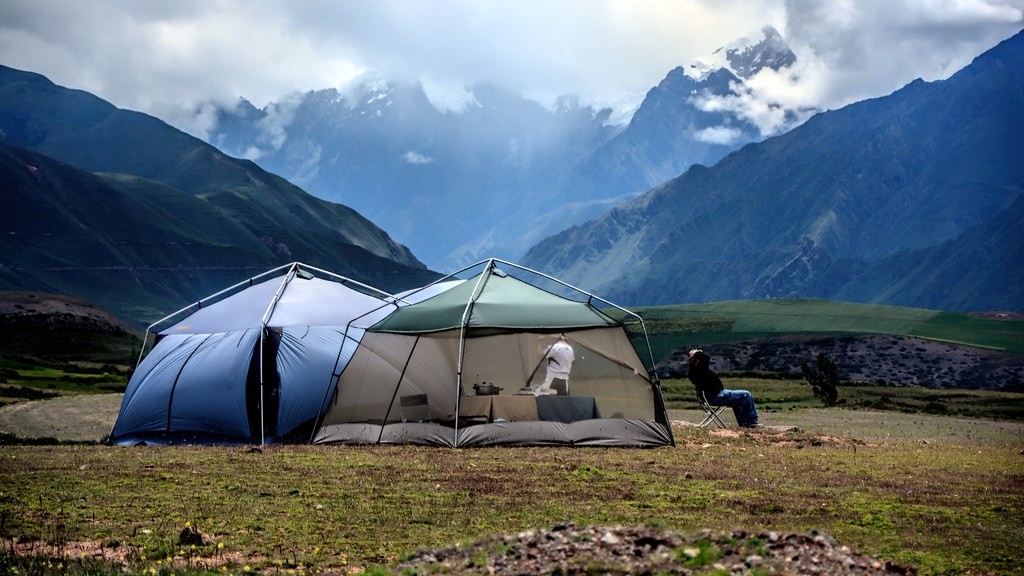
[473, 381, 504, 396]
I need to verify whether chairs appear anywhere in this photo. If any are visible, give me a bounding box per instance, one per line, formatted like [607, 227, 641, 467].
[694, 385, 727, 428]
[400, 393, 434, 423]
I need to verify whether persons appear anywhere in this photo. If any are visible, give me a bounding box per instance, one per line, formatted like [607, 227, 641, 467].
[685, 344, 764, 428]
[535, 332, 577, 398]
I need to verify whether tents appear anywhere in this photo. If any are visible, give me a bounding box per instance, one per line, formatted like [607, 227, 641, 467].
[380, 278, 466, 308]
[107, 260, 412, 447]
[306, 256, 674, 446]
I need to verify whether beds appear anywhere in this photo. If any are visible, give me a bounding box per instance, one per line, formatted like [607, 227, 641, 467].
[460, 394, 599, 423]
